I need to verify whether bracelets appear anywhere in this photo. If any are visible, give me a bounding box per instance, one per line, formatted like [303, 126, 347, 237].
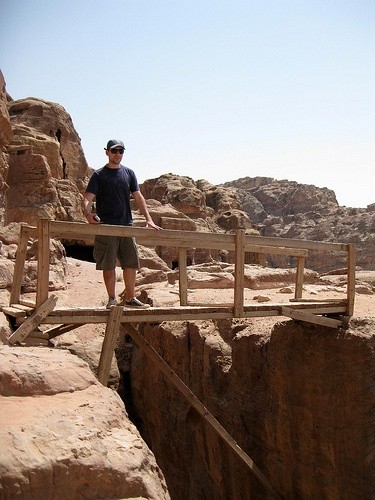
[86, 214, 89, 218]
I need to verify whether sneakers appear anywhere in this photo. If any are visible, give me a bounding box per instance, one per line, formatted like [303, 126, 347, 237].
[106, 297, 117, 309]
[124, 297, 150, 308]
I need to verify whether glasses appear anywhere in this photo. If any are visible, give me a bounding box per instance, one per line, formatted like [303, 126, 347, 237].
[111, 149, 124, 154]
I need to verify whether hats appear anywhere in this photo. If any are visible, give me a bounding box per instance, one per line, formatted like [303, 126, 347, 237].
[104, 139, 125, 151]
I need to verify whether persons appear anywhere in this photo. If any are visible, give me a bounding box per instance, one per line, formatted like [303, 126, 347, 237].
[82, 139, 163, 309]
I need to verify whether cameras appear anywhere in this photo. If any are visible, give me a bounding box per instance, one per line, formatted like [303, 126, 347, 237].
[94, 215, 101, 221]
